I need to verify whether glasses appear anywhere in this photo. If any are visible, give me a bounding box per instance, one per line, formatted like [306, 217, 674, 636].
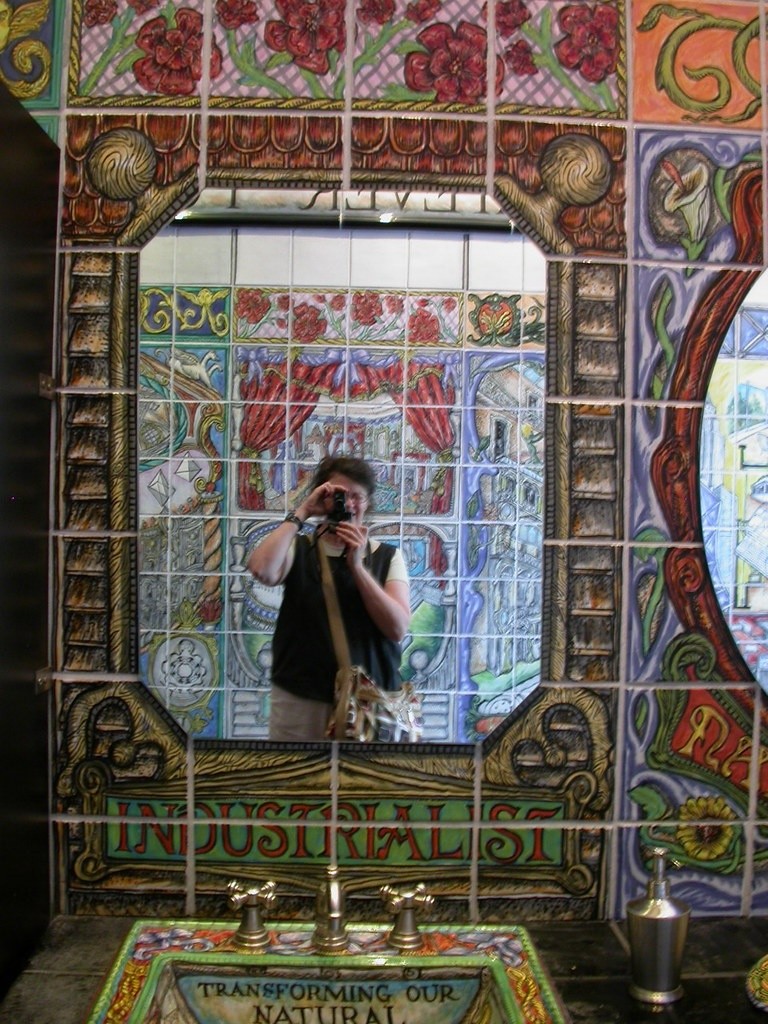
[345, 492, 371, 505]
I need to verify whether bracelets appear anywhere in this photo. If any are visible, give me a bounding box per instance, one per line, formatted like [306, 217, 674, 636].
[286, 513, 303, 533]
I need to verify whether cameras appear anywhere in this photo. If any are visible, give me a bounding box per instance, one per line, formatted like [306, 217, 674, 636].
[326, 490, 352, 534]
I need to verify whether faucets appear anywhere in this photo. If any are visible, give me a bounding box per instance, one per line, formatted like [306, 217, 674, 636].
[313, 865, 350, 951]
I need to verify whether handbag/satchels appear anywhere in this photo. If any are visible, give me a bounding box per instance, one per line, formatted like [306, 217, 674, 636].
[324, 664, 423, 743]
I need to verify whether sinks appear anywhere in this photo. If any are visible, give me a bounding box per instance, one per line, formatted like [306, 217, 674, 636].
[85, 920, 572, 1024]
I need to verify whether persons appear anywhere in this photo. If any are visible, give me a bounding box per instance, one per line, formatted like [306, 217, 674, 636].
[249, 457, 410, 742]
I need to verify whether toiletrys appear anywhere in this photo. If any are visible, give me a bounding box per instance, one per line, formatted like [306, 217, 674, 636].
[623, 846, 686, 1002]
